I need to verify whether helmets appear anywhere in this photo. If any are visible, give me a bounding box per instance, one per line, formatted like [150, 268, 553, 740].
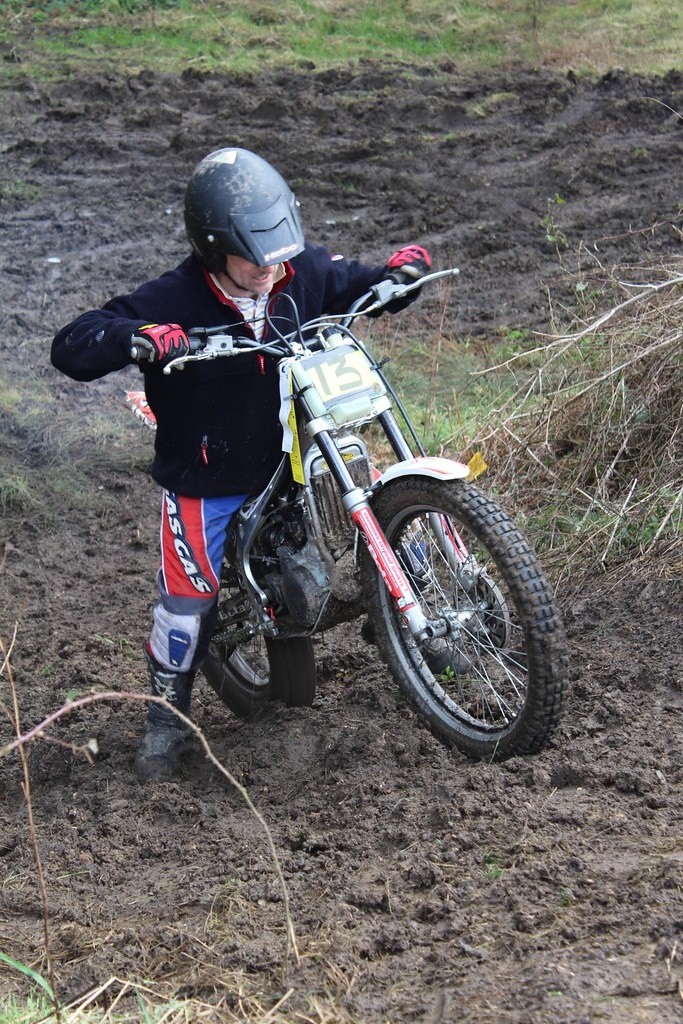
[182, 147, 305, 273]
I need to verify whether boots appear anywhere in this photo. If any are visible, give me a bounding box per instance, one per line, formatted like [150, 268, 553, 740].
[360, 563, 475, 677]
[133, 643, 196, 783]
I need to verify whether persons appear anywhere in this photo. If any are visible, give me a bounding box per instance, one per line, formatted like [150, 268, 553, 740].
[49, 147, 432, 788]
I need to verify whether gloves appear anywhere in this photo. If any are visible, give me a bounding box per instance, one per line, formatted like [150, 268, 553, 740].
[131, 323, 190, 371]
[384, 244, 432, 308]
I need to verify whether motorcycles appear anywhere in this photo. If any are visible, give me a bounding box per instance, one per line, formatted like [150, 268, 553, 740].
[134, 257, 563, 763]
